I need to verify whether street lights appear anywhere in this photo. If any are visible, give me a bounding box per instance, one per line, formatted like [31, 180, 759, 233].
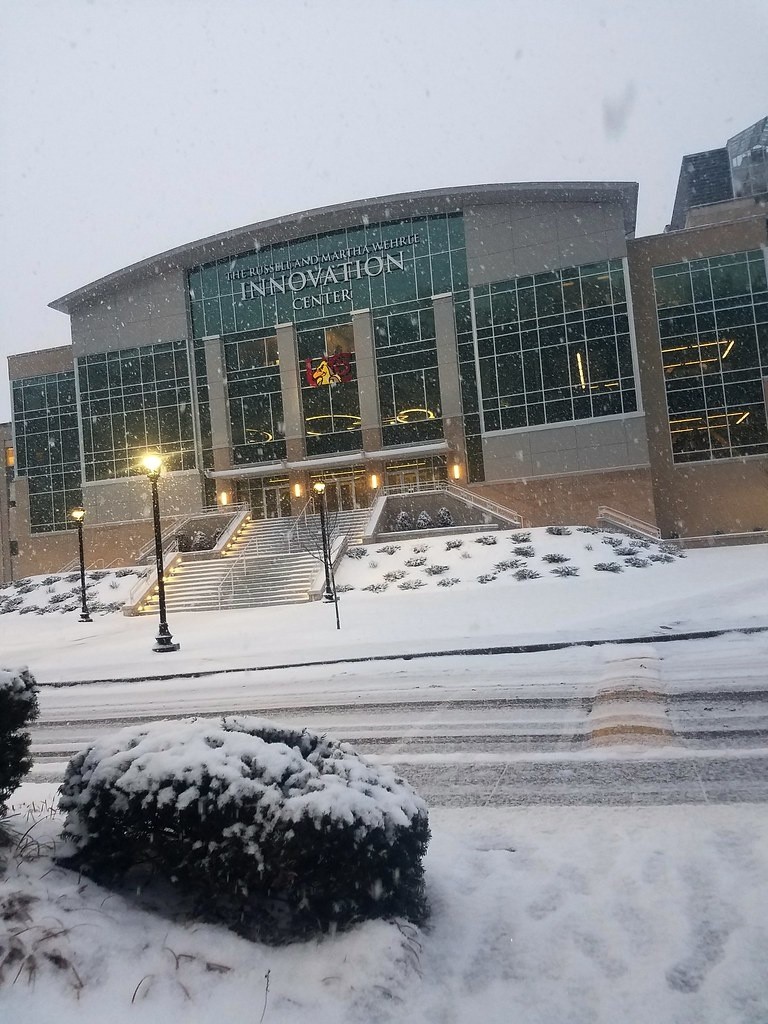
[71, 508, 93, 622]
[141, 453, 181, 652]
[314, 482, 335, 603]
